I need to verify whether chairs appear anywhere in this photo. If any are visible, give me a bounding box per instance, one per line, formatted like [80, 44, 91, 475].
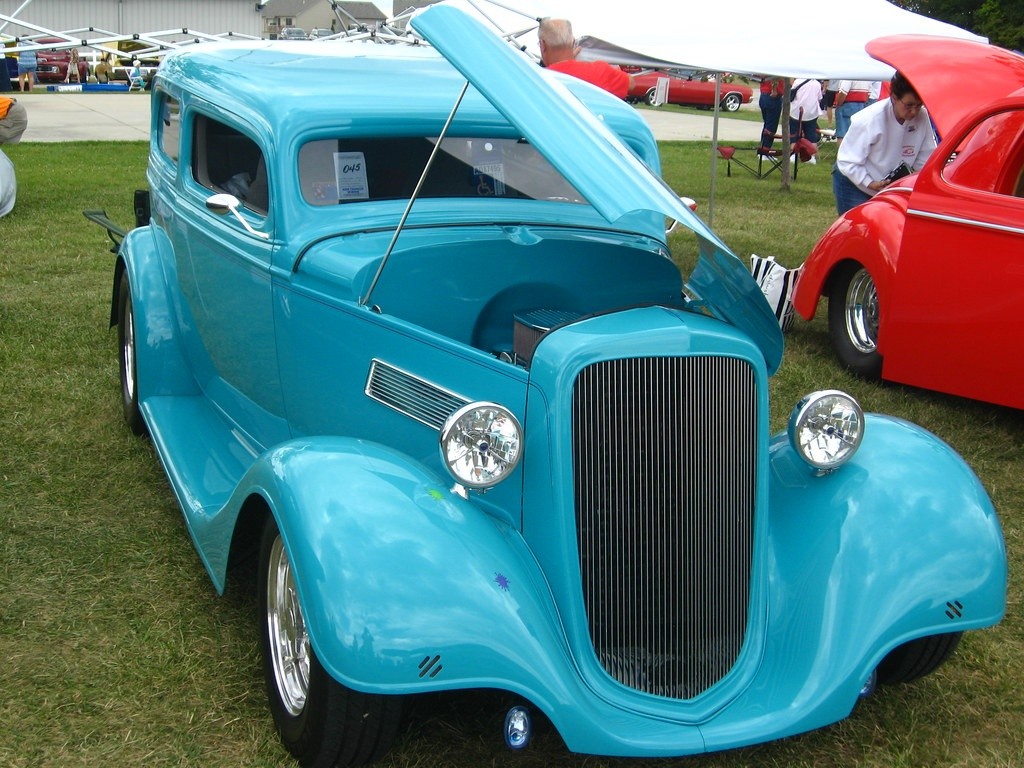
[718, 105, 837, 180]
[124, 67, 143, 92]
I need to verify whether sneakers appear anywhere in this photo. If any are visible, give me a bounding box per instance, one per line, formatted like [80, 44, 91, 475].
[805, 155, 817, 165]
[790, 153, 795, 162]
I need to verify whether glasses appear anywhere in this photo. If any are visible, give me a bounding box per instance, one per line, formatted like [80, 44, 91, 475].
[898, 99, 924, 109]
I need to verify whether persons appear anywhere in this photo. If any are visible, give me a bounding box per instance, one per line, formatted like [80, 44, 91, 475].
[759, 76, 882, 164]
[0, 96, 28, 144]
[537, 16, 631, 103]
[64, 48, 80, 84]
[130, 60, 145, 91]
[832, 70, 938, 213]
[19, 34, 38, 93]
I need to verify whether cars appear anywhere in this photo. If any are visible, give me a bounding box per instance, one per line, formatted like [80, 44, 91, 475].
[94, 40, 161, 86]
[626, 68, 755, 113]
[33, 38, 91, 86]
[80, 0, 1012, 768]
[790, 35, 1024, 410]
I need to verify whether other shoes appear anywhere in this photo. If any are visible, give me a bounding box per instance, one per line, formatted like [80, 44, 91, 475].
[140, 89, 146, 92]
[758, 153, 777, 161]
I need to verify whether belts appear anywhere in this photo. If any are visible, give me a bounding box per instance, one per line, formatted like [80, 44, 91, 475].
[7, 98, 16, 114]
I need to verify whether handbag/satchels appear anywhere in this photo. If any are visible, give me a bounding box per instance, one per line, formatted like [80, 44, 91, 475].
[749, 253, 805, 333]
[790, 89, 797, 101]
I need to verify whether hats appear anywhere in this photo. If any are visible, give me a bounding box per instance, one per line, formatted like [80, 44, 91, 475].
[133, 60, 141, 67]
